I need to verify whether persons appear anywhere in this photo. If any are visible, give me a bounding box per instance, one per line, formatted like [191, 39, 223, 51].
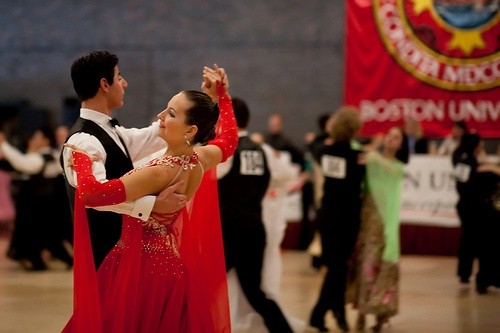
[59, 50, 229, 271]
[0, 64, 500, 333]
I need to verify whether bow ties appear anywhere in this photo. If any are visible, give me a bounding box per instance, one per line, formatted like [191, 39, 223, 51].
[109, 118, 120, 127]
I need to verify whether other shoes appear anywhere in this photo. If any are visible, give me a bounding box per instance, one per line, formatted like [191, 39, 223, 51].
[338, 320, 348, 332]
[311, 321, 328, 332]
